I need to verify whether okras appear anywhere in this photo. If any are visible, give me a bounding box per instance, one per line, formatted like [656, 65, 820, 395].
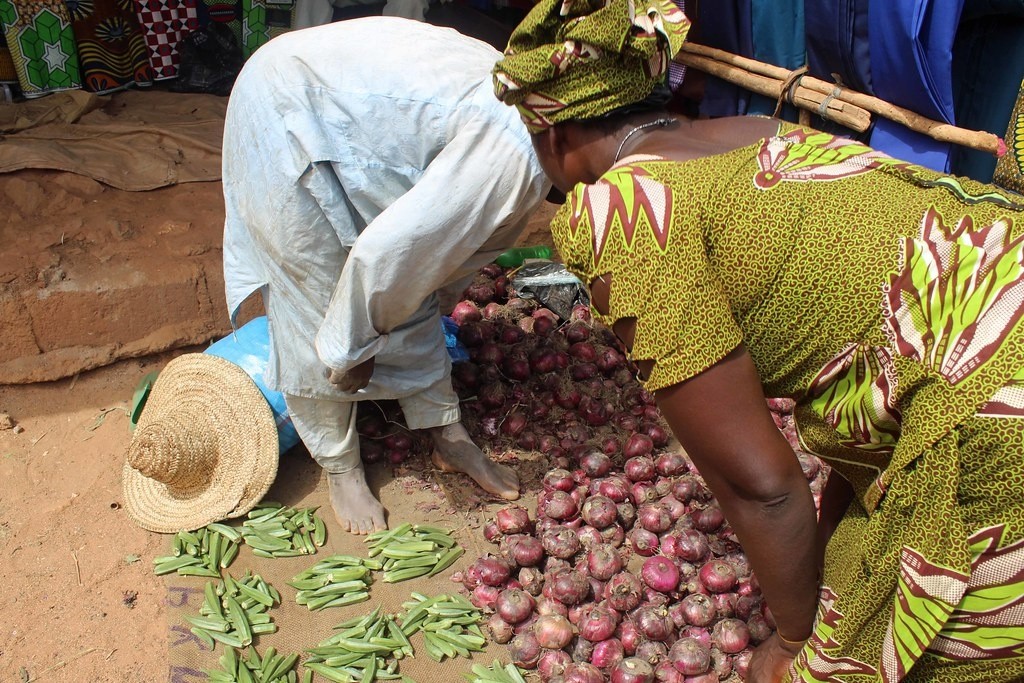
[155, 504, 528, 683]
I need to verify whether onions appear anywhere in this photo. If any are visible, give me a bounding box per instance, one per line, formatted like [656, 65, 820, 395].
[363, 261, 833, 683]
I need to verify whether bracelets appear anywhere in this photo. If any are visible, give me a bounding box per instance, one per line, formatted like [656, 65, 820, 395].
[774, 627, 810, 649]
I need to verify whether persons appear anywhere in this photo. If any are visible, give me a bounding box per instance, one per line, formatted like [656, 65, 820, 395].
[219, 15, 566, 536]
[489, 1, 1024, 683]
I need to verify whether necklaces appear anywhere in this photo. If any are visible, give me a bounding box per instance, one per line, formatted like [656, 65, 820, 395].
[613, 114, 678, 163]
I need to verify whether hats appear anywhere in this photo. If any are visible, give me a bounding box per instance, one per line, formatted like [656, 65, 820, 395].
[122, 353, 279, 534]
